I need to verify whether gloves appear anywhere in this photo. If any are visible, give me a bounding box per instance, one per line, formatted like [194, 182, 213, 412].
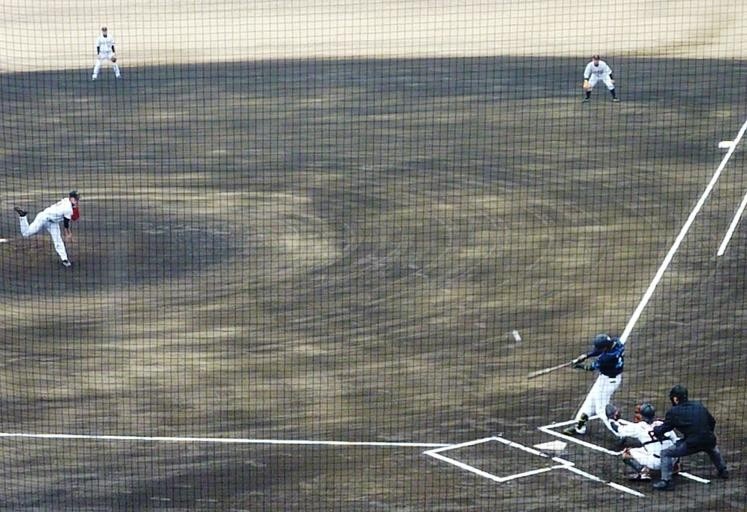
[573, 354, 587, 369]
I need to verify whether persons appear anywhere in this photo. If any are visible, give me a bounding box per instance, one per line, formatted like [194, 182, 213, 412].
[14, 191, 81, 267]
[607, 400, 682, 481]
[563, 333, 627, 445]
[91, 27, 124, 81]
[581, 54, 621, 103]
[652, 385, 728, 488]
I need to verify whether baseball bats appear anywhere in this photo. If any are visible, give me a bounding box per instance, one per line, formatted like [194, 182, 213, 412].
[528, 356, 586, 380]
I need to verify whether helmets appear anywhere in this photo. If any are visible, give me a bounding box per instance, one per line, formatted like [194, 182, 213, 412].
[670, 385, 688, 406]
[640, 404, 655, 417]
[594, 334, 612, 348]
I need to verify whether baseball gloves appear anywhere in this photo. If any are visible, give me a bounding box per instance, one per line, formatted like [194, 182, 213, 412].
[606, 404, 623, 421]
[111, 54, 117, 62]
[584, 81, 592, 90]
[63, 229, 73, 243]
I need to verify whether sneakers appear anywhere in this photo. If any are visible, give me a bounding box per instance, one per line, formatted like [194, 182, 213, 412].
[634, 472, 652, 480]
[713, 469, 729, 478]
[63, 260, 71, 267]
[653, 480, 673, 490]
[564, 425, 586, 434]
[15, 207, 26, 216]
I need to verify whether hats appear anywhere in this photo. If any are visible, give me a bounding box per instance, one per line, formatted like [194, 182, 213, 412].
[70, 191, 80, 199]
